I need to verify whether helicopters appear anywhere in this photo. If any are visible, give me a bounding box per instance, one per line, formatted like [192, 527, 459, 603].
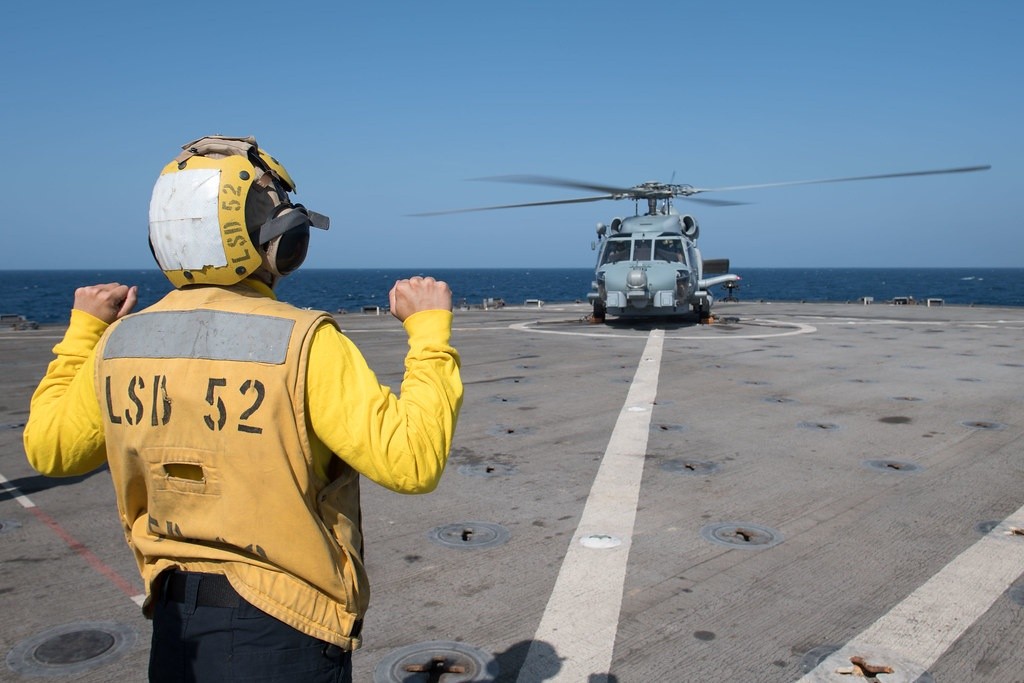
[406, 162, 993, 324]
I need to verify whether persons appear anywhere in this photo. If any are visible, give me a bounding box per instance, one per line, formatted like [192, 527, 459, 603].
[22, 135, 464, 683]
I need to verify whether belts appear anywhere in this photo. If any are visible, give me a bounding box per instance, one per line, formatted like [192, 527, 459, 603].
[155, 571, 259, 616]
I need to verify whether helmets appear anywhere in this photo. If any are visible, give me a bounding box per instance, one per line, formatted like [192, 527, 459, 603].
[148, 135, 329, 289]
